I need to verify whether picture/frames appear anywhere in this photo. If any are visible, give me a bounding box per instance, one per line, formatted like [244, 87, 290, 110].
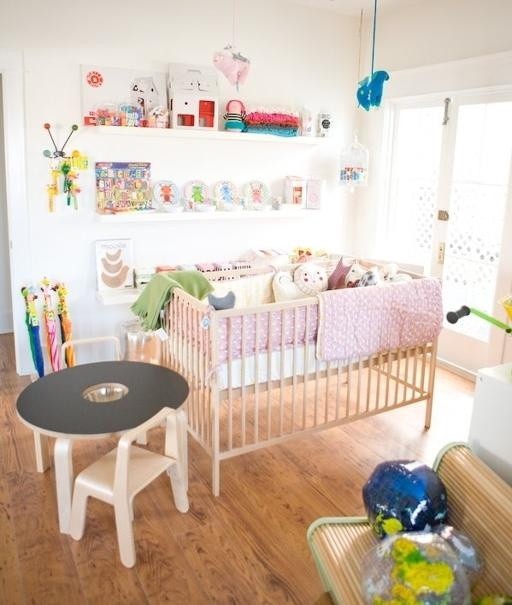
[95, 238, 136, 290]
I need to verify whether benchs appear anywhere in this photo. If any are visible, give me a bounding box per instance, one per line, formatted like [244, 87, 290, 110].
[308, 440, 512, 605]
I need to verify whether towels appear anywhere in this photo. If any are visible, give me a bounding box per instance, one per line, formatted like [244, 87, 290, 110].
[130, 269, 215, 335]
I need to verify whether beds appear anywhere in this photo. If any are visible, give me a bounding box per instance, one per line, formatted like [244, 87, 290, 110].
[158, 254, 443, 497]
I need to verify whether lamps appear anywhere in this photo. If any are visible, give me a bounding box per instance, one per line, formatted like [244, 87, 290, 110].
[355, 10, 373, 115]
[370, 1, 391, 113]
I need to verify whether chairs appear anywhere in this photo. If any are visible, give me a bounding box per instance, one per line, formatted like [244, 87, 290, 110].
[69, 406, 192, 569]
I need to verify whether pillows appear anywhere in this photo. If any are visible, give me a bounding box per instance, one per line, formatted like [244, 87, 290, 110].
[270, 271, 309, 305]
[198, 272, 274, 311]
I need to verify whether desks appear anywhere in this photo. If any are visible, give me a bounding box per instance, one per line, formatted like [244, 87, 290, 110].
[17, 358, 192, 535]
[468, 360, 512, 486]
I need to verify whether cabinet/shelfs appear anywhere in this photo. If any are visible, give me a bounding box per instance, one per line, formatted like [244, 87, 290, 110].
[87, 125, 327, 305]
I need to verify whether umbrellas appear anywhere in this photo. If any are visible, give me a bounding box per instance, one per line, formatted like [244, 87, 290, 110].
[54, 280, 76, 368]
[22, 283, 45, 381]
[39, 276, 62, 374]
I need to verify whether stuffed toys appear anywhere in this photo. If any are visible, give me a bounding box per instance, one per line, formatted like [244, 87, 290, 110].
[223, 100, 248, 132]
[269, 255, 411, 309]
